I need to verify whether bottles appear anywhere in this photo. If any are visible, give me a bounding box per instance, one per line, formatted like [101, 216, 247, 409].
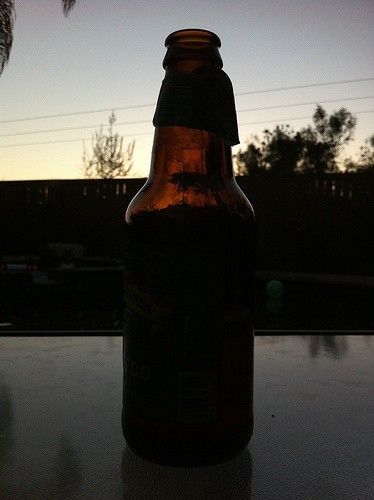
[119, 27, 262, 468]
